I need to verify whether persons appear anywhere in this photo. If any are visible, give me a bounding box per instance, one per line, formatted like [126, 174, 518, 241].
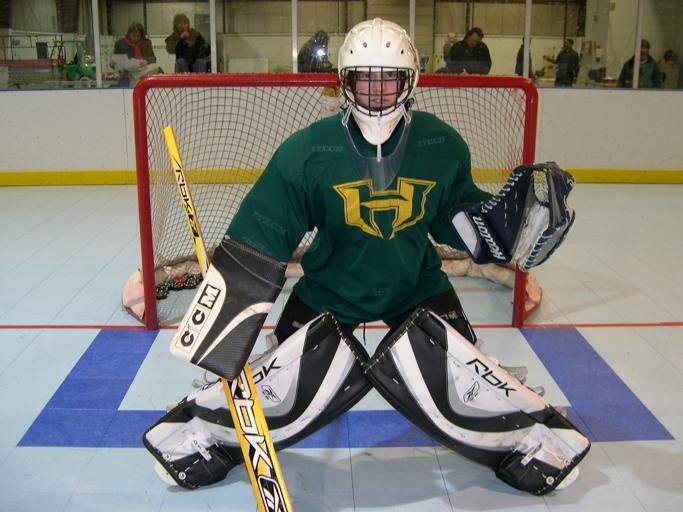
[542, 39, 579, 87]
[450, 27, 493, 74]
[658, 49, 678, 66]
[616, 37, 662, 89]
[163, 14, 210, 74]
[515, 35, 536, 83]
[151, 16, 579, 493]
[111, 23, 156, 87]
[296, 30, 329, 73]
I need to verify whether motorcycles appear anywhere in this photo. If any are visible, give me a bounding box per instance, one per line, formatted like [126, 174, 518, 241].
[299, 39, 332, 73]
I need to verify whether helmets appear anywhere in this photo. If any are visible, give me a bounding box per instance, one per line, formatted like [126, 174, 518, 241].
[336, 19, 424, 129]
